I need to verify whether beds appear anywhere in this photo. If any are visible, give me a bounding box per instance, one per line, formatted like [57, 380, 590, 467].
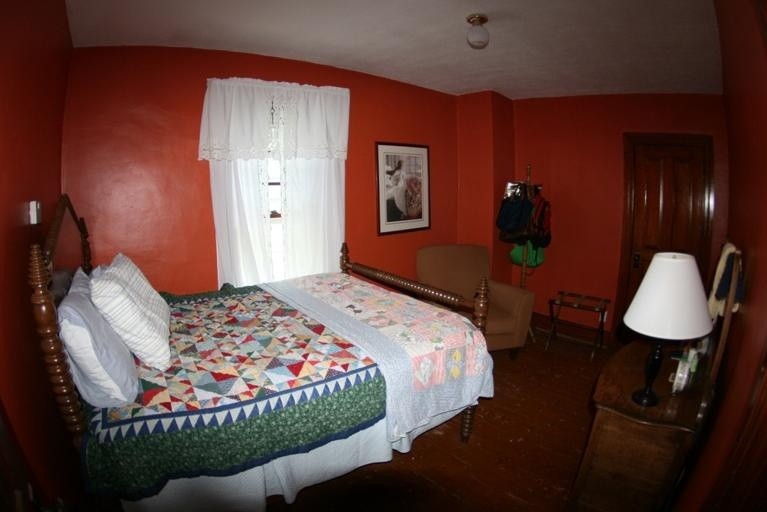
[27, 194, 490, 512]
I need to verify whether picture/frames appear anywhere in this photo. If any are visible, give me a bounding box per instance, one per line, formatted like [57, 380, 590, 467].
[374, 140, 431, 236]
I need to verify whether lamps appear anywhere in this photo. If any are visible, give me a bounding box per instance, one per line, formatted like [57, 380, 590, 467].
[622, 252, 714, 410]
[465, 13, 491, 48]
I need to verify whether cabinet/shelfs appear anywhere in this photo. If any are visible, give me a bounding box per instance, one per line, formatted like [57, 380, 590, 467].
[568, 339, 700, 510]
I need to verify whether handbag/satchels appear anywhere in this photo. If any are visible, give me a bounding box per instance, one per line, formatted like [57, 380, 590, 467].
[496, 182, 551, 268]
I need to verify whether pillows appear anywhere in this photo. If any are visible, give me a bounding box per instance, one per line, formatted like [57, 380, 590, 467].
[54, 252, 173, 412]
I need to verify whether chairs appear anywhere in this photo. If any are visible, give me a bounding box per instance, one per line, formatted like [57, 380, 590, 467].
[414, 242, 535, 361]
